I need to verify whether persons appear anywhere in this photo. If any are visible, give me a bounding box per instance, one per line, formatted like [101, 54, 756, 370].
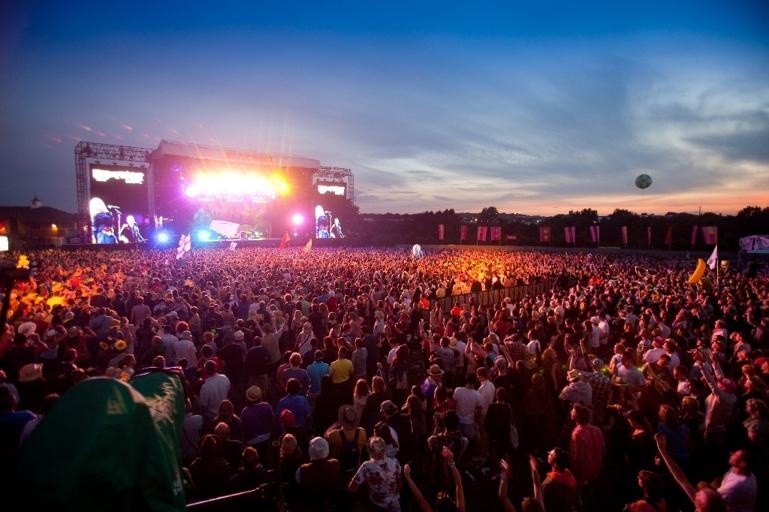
[2, 232, 769, 511]
[118, 214, 141, 242]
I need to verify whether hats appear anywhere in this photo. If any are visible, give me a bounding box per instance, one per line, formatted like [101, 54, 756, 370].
[246, 386, 262, 402]
[426, 364, 445, 377]
[337, 404, 360, 428]
[378, 400, 392, 416]
[309, 436, 329, 461]
[18, 363, 43, 382]
[566, 368, 580, 382]
[241, 447, 260, 462]
[366, 436, 386, 462]
